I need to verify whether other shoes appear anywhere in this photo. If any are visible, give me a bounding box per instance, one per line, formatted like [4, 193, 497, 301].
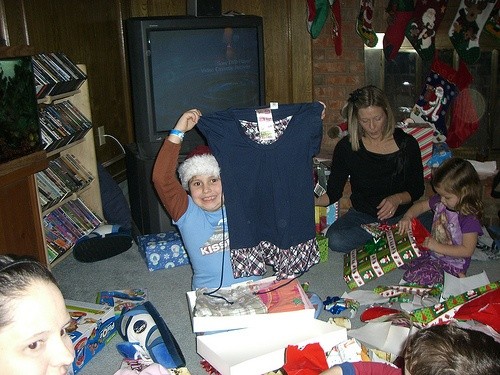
[73, 223, 135, 262]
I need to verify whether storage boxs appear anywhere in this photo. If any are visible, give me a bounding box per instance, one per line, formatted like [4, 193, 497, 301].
[343, 221, 420, 290]
[138, 231, 190, 271]
[316, 232, 328, 264]
[96, 289, 148, 316]
[187, 276, 347, 375]
[64, 299, 118, 375]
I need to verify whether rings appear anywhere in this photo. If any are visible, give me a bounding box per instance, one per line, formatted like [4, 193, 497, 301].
[389, 211, 392, 214]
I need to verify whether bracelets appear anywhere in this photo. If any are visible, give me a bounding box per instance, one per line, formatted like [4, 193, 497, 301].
[170, 129, 184, 141]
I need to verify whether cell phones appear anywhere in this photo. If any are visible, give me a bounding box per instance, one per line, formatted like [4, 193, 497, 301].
[314, 182, 326, 196]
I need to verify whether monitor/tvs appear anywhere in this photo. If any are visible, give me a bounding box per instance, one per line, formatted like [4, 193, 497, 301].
[0, 56, 42, 165]
[120, 16, 267, 157]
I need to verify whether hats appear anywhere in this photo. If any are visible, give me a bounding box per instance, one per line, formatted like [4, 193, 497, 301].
[178, 146, 220, 192]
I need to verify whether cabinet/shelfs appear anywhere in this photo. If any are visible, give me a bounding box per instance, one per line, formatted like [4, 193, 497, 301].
[29, 52, 103, 271]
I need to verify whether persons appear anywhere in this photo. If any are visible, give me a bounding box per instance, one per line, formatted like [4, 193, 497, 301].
[153, 102, 327, 291]
[398, 158, 486, 288]
[320, 323, 500, 375]
[314, 85, 425, 253]
[0, 253, 170, 375]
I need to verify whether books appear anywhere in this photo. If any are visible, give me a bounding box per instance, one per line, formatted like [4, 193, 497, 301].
[33, 53, 94, 265]
